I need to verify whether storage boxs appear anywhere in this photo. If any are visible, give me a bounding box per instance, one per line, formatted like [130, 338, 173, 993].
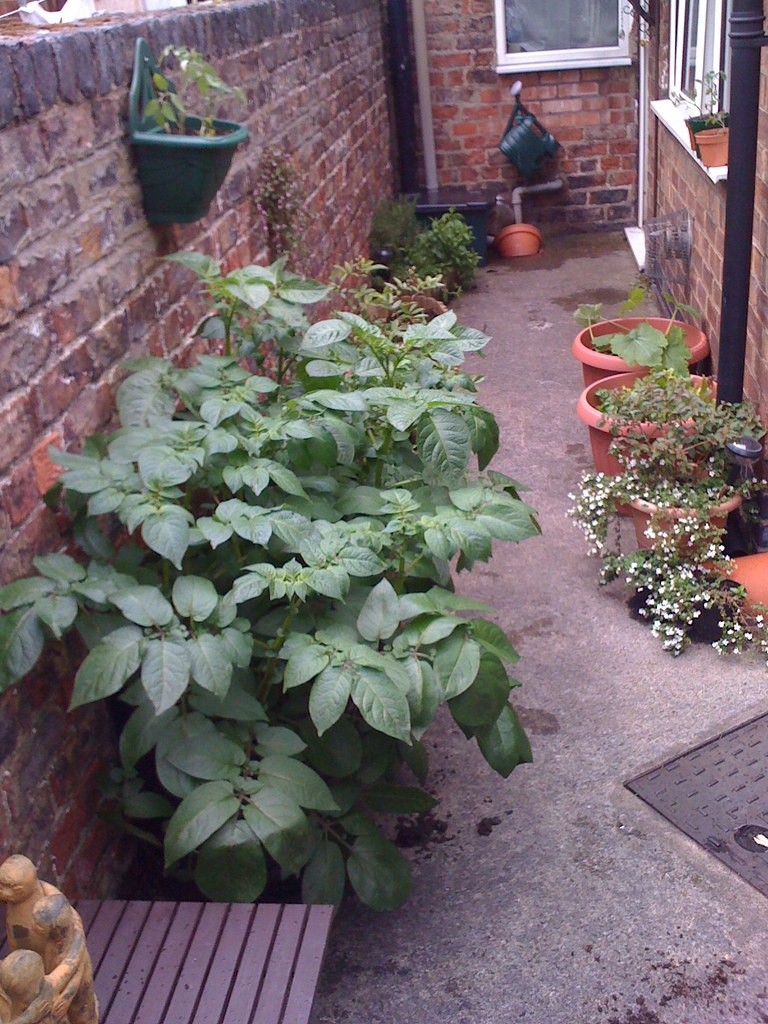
[413, 191, 500, 263]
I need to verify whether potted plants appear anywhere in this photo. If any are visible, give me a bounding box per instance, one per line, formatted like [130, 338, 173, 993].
[0, 190, 544, 916]
[671, 70, 729, 167]
[127, 35, 248, 224]
[571, 284, 718, 516]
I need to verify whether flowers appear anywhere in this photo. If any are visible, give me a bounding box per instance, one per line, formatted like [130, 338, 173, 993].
[567, 445, 768, 658]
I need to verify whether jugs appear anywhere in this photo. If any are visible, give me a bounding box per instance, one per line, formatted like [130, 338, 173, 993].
[497, 79, 560, 181]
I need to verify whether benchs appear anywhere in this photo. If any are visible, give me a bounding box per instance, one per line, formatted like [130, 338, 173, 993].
[61, 898, 334, 1024]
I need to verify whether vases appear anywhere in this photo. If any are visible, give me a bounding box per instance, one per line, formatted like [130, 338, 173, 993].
[629, 490, 743, 562]
[692, 578, 746, 641]
[494, 223, 542, 257]
